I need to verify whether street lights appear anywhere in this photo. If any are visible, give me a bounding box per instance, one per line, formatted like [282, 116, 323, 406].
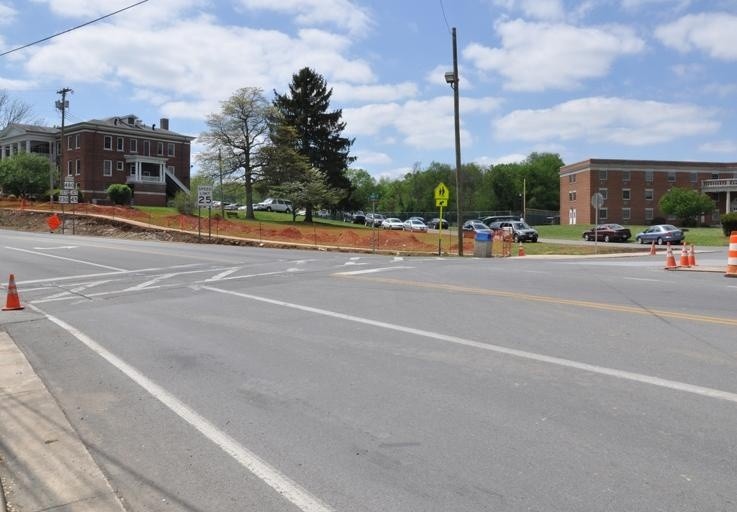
[443, 27, 466, 256]
[55, 86, 75, 190]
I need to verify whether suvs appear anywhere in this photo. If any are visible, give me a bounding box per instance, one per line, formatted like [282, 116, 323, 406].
[364, 213, 385, 227]
[343, 210, 365, 225]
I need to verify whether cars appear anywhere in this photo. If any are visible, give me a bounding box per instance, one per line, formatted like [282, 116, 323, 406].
[212, 196, 331, 218]
[464, 213, 538, 244]
[635, 224, 686, 245]
[409, 216, 427, 225]
[583, 223, 632, 244]
[402, 219, 428, 233]
[381, 217, 403, 230]
[427, 218, 448, 230]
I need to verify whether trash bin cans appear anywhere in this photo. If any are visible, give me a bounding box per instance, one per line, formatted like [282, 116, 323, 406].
[473, 232, 493, 258]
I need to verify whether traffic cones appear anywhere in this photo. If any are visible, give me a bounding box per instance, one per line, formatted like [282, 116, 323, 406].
[3, 272, 24, 311]
[665, 242, 677, 270]
[19, 199, 26, 209]
[687, 244, 697, 267]
[725, 231, 737, 276]
[517, 241, 526, 256]
[679, 242, 689, 268]
[649, 238, 657, 255]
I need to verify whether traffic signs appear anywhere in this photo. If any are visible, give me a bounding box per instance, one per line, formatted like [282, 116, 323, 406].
[198, 186, 213, 206]
